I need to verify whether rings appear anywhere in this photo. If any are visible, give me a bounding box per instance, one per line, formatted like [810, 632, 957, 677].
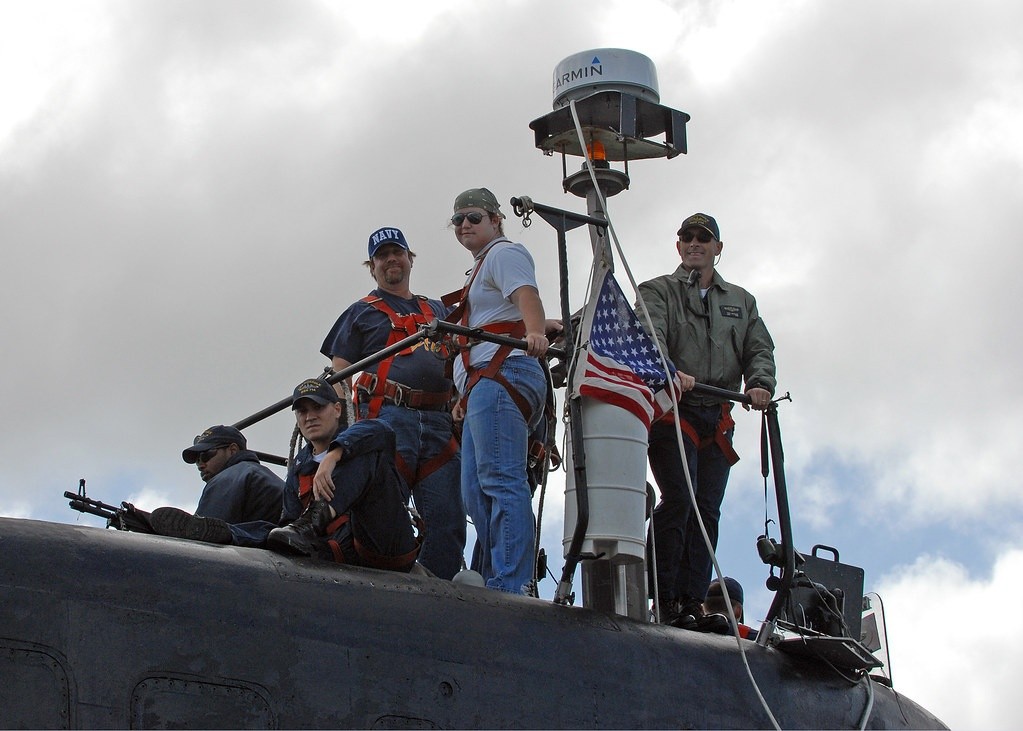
[762, 400, 766, 402]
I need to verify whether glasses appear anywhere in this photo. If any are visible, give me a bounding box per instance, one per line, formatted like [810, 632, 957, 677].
[679, 230, 713, 243]
[450, 211, 489, 226]
[198, 448, 219, 465]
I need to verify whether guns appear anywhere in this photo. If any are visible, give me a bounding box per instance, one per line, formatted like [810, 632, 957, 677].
[63, 478, 153, 534]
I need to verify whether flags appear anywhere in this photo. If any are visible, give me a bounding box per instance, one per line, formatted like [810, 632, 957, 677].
[567, 264, 683, 431]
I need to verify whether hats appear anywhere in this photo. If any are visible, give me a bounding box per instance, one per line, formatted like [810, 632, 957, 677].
[709, 576, 744, 624]
[291, 379, 339, 412]
[368, 227, 410, 257]
[182, 425, 246, 465]
[677, 213, 720, 242]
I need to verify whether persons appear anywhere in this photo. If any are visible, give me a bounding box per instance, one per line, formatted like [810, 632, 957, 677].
[321, 228, 564, 580]
[634, 214, 777, 635]
[452, 187, 549, 597]
[183, 425, 285, 524]
[703, 577, 759, 641]
[149, 379, 421, 574]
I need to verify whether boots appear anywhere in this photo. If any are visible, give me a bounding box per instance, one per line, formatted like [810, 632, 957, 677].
[267, 495, 334, 558]
[149, 507, 232, 542]
[653, 597, 729, 634]
